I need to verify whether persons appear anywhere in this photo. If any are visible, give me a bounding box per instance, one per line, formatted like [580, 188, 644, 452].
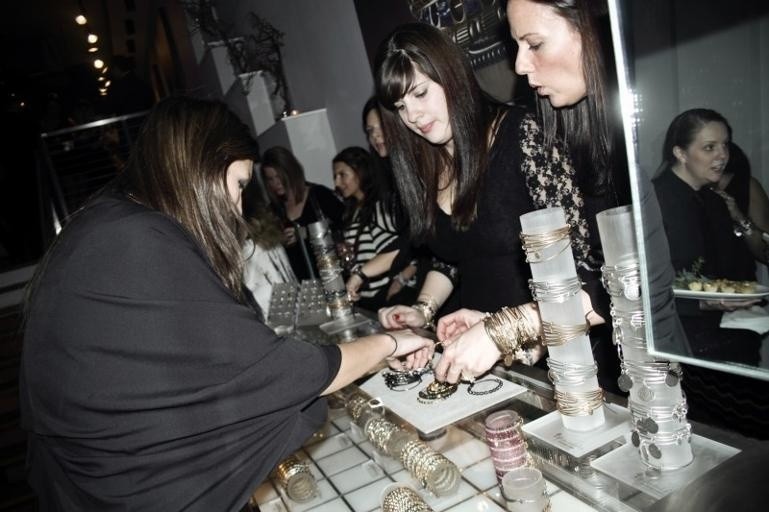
[375, 26, 524, 332]
[655, 108, 762, 367]
[330, 146, 403, 304]
[362, 93, 426, 307]
[261, 146, 341, 282]
[708, 145, 769, 268]
[434, 0, 639, 388]
[17, 97, 435, 512]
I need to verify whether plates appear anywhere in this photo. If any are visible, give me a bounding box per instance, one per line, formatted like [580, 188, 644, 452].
[673, 283, 764, 300]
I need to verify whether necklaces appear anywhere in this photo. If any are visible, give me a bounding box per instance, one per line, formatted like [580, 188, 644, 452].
[436, 177, 452, 192]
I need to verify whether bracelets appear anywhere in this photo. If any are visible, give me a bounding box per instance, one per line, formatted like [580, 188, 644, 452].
[486, 407, 553, 512]
[346, 390, 460, 497]
[520, 225, 573, 265]
[611, 327, 647, 354]
[605, 302, 646, 330]
[630, 422, 694, 462]
[382, 483, 434, 511]
[468, 378, 503, 396]
[734, 217, 752, 238]
[483, 301, 538, 368]
[540, 320, 588, 347]
[552, 387, 606, 417]
[306, 228, 353, 312]
[617, 358, 685, 402]
[598, 253, 640, 302]
[409, 293, 438, 331]
[270, 453, 318, 504]
[392, 273, 408, 287]
[625, 389, 691, 432]
[546, 356, 601, 389]
[527, 274, 583, 304]
[353, 268, 368, 282]
[380, 332, 398, 359]
[386, 360, 457, 405]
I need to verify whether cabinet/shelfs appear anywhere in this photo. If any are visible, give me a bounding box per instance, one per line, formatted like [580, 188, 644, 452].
[248, 303, 768, 512]
[180, 24, 340, 192]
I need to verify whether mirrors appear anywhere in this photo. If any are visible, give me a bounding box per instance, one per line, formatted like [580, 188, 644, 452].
[608, 1, 769, 381]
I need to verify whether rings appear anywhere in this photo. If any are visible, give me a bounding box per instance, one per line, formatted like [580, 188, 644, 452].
[461, 372, 475, 384]
